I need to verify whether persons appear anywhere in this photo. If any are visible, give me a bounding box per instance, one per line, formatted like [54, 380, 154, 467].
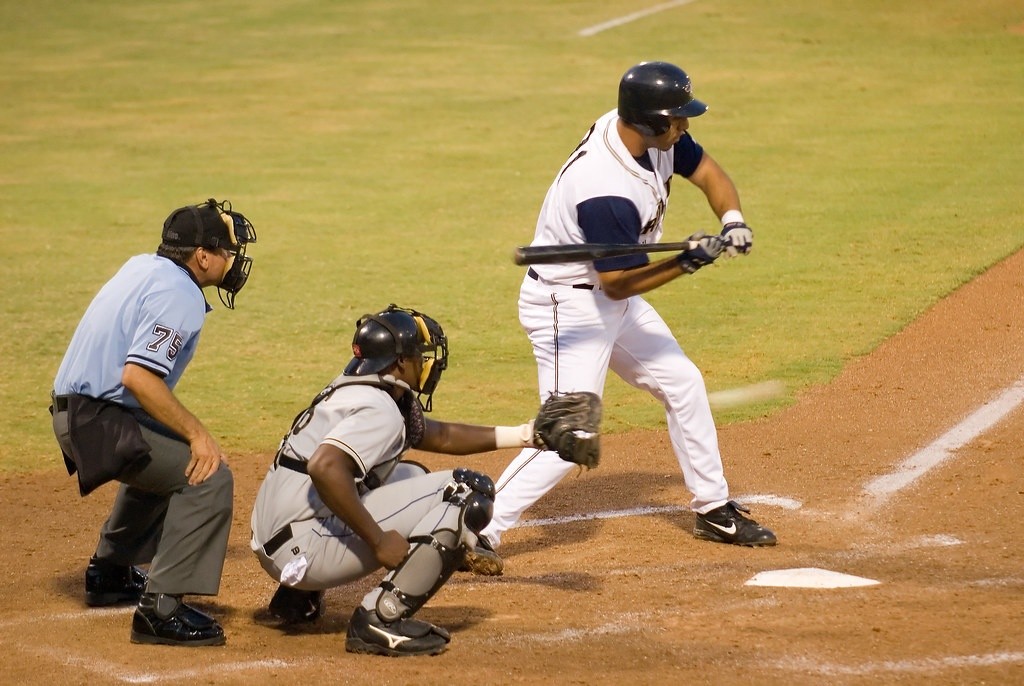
[453, 60, 778, 573]
[251, 305, 602, 655]
[51, 198, 257, 646]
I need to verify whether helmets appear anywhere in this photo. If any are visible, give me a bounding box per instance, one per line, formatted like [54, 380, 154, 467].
[343, 309, 419, 377]
[618, 61, 709, 136]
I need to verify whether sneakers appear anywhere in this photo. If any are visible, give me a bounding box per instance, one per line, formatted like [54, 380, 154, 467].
[345, 606, 450, 656]
[457, 535, 504, 575]
[693, 501, 777, 546]
[269, 584, 321, 622]
[85, 556, 149, 606]
[130, 593, 227, 646]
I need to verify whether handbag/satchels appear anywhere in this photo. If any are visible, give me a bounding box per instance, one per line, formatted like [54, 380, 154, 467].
[68, 393, 152, 497]
[49, 405, 77, 476]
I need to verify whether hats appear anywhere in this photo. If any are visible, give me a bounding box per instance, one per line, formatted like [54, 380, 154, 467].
[162, 206, 234, 246]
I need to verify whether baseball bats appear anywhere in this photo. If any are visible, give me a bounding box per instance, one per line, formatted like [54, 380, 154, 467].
[515, 240, 699, 266]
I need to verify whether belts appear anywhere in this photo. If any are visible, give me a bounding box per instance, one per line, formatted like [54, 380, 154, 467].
[263, 524, 293, 557]
[56, 396, 69, 412]
[528, 267, 601, 290]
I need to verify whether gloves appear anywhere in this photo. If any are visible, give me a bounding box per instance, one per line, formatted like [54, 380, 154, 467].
[678, 232, 726, 275]
[720, 222, 754, 257]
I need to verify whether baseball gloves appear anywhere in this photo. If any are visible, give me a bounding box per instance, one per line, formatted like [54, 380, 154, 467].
[533, 391, 602, 469]
